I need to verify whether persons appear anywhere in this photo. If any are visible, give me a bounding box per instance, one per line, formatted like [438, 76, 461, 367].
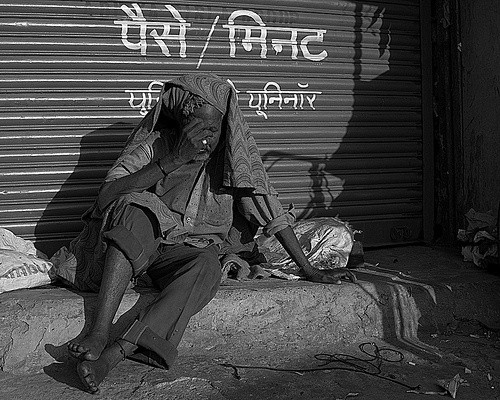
[67, 71, 360, 395]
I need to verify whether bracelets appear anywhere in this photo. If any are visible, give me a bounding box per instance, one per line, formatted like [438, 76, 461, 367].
[157, 157, 168, 176]
[298, 262, 310, 273]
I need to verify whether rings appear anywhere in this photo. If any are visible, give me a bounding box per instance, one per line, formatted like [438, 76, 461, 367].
[202, 139, 207, 145]
